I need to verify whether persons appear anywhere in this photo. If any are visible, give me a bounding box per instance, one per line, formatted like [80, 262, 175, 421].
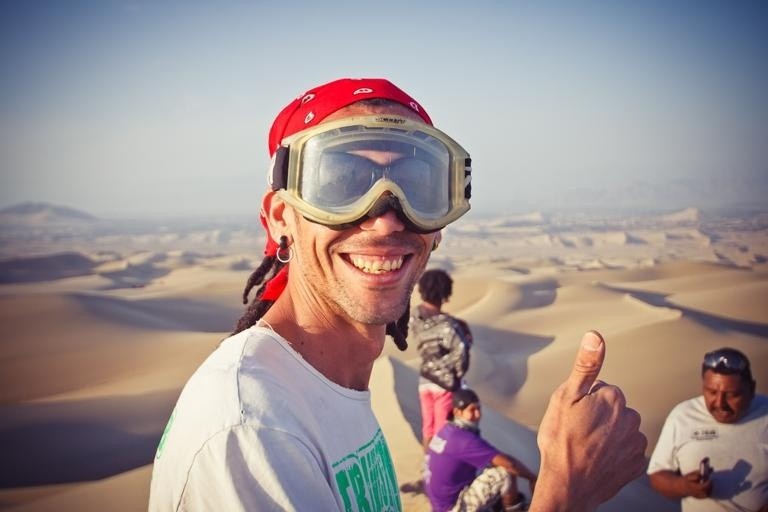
[410, 266, 475, 485]
[422, 386, 536, 511]
[148, 75, 650, 512]
[643, 346, 767, 511]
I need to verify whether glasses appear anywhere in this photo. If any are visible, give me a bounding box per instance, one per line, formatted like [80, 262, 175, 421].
[268, 113, 472, 234]
[704, 351, 747, 372]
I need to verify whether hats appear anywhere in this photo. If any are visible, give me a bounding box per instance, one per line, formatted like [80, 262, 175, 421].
[446, 388, 478, 422]
